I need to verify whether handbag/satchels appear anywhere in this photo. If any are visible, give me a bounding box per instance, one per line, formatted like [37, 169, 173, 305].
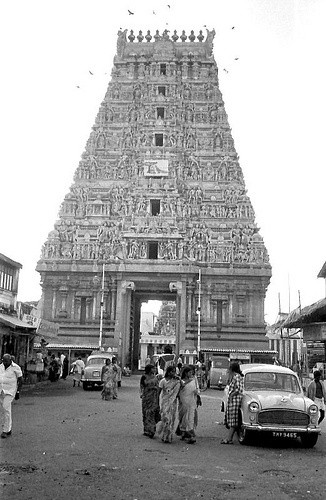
[197, 394, 201, 406]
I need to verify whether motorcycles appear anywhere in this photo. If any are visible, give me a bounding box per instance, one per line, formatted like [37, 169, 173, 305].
[207, 356, 230, 391]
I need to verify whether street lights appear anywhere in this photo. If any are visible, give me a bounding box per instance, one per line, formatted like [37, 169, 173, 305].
[195, 268, 201, 360]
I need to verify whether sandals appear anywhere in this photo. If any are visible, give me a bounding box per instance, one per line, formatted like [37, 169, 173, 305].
[221, 439, 233, 444]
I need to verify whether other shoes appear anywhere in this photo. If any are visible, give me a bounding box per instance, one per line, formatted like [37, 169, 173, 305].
[1, 431, 11, 437]
[189, 439, 195, 443]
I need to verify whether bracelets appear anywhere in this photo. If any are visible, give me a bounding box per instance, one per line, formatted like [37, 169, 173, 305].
[17, 391, 19, 393]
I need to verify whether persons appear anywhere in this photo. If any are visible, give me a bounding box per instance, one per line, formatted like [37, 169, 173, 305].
[123, 364, 129, 376]
[145, 355, 151, 367]
[307, 371, 326, 434]
[139, 363, 202, 443]
[220, 363, 244, 444]
[71, 357, 85, 387]
[44, 354, 69, 382]
[0, 353, 23, 438]
[101, 357, 122, 400]
[177, 354, 183, 364]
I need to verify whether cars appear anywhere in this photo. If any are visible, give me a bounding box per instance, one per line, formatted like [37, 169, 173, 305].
[80, 353, 122, 390]
[221, 363, 321, 450]
